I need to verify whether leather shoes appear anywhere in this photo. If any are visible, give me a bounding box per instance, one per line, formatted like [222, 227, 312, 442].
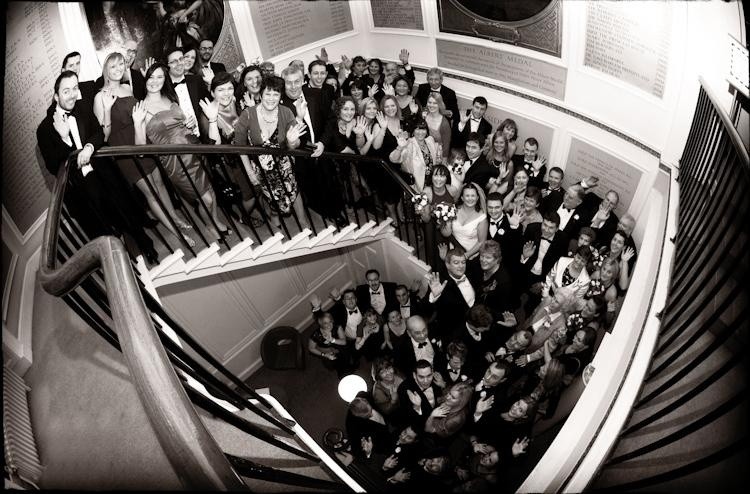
[145, 247, 159, 264]
[328, 211, 348, 227]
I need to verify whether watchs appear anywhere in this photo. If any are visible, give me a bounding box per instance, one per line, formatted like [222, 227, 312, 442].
[404, 62, 408, 65]
[84, 145, 93, 151]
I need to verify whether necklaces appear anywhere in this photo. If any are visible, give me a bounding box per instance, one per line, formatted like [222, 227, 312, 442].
[491, 160, 502, 168]
[260, 107, 279, 123]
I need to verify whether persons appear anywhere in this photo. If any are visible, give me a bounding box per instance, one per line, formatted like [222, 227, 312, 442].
[308, 313, 346, 377]
[36, 70, 160, 265]
[382, 78, 423, 125]
[473, 360, 509, 428]
[370, 358, 407, 417]
[290, 48, 338, 83]
[452, 96, 492, 160]
[544, 326, 598, 419]
[451, 466, 484, 494]
[425, 92, 452, 164]
[483, 118, 518, 161]
[469, 435, 504, 484]
[180, 46, 215, 102]
[514, 137, 551, 190]
[350, 82, 379, 118]
[341, 56, 375, 99]
[488, 161, 530, 203]
[389, 120, 442, 241]
[260, 62, 282, 78]
[496, 165, 528, 192]
[355, 307, 387, 382]
[484, 330, 532, 384]
[596, 230, 634, 290]
[234, 64, 265, 117]
[93, 52, 196, 247]
[359, 97, 393, 216]
[132, 63, 232, 244]
[384, 456, 453, 485]
[235, 76, 313, 236]
[581, 296, 613, 331]
[199, 39, 225, 100]
[310, 95, 369, 207]
[282, 66, 348, 228]
[325, 75, 338, 112]
[302, 60, 335, 152]
[199, 72, 258, 202]
[486, 192, 526, 311]
[433, 342, 476, 395]
[538, 245, 591, 312]
[517, 210, 570, 320]
[445, 181, 492, 274]
[383, 311, 416, 369]
[163, 47, 214, 145]
[557, 184, 586, 232]
[417, 165, 459, 278]
[523, 359, 566, 422]
[61, 52, 160, 229]
[397, 360, 445, 439]
[516, 279, 569, 367]
[328, 269, 422, 315]
[446, 149, 465, 182]
[570, 176, 620, 229]
[379, 423, 422, 474]
[482, 396, 535, 467]
[400, 316, 447, 379]
[345, 392, 396, 458]
[502, 180, 544, 235]
[462, 132, 492, 194]
[420, 247, 482, 334]
[416, 68, 457, 113]
[309, 293, 364, 368]
[437, 240, 522, 316]
[424, 382, 473, 438]
[372, 95, 408, 227]
[454, 304, 518, 373]
[537, 167, 568, 217]
[485, 132, 513, 185]
[382, 285, 423, 320]
[568, 226, 599, 264]
[385, 51, 415, 96]
[96, 40, 183, 210]
[343, 58, 385, 107]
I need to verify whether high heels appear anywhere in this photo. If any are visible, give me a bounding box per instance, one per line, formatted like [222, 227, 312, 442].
[385, 212, 400, 228]
[218, 235, 226, 244]
[399, 216, 406, 223]
[221, 226, 232, 235]
[278, 223, 282, 230]
[179, 233, 196, 247]
[176, 220, 193, 229]
[240, 216, 265, 228]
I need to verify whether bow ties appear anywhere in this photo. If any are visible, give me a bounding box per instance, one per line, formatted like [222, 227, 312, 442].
[446, 368, 458, 375]
[429, 89, 439, 94]
[562, 204, 572, 214]
[371, 291, 379, 296]
[540, 235, 554, 244]
[401, 305, 411, 308]
[456, 278, 466, 286]
[502, 342, 508, 354]
[170, 79, 187, 88]
[61, 110, 74, 119]
[488, 221, 501, 226]
[471, 117, 480, 124]
[422, 385, 431, 392]
[349, 308, 360, 315]
[523, 159, 534, 167]
[465, 158, 473, 165]
[418, 342, 429, 348]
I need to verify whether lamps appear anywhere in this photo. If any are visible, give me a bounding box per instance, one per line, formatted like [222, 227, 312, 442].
[337, 374, 371, 403]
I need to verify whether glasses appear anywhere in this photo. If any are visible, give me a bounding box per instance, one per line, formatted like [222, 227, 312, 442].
[367, 278, 379, 283]
[168, 55, 183, 66]
[369, 64, 380, 70]
[200, 46, 213, 52]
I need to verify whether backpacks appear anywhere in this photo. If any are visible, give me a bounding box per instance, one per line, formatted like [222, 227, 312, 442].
[590, 201, 637, 278]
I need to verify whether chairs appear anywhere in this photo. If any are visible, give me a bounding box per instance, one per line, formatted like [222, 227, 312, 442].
[260, 326, 306, 372]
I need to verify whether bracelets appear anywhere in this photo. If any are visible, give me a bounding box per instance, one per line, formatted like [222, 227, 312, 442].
[102, 123, 112, 127]
[320, 351, 328, 359]
[442, 386, 447, 390]
[208, 119, 218, 123]
[494, 180, 502, 186]
[471, 439, 477, 443]
